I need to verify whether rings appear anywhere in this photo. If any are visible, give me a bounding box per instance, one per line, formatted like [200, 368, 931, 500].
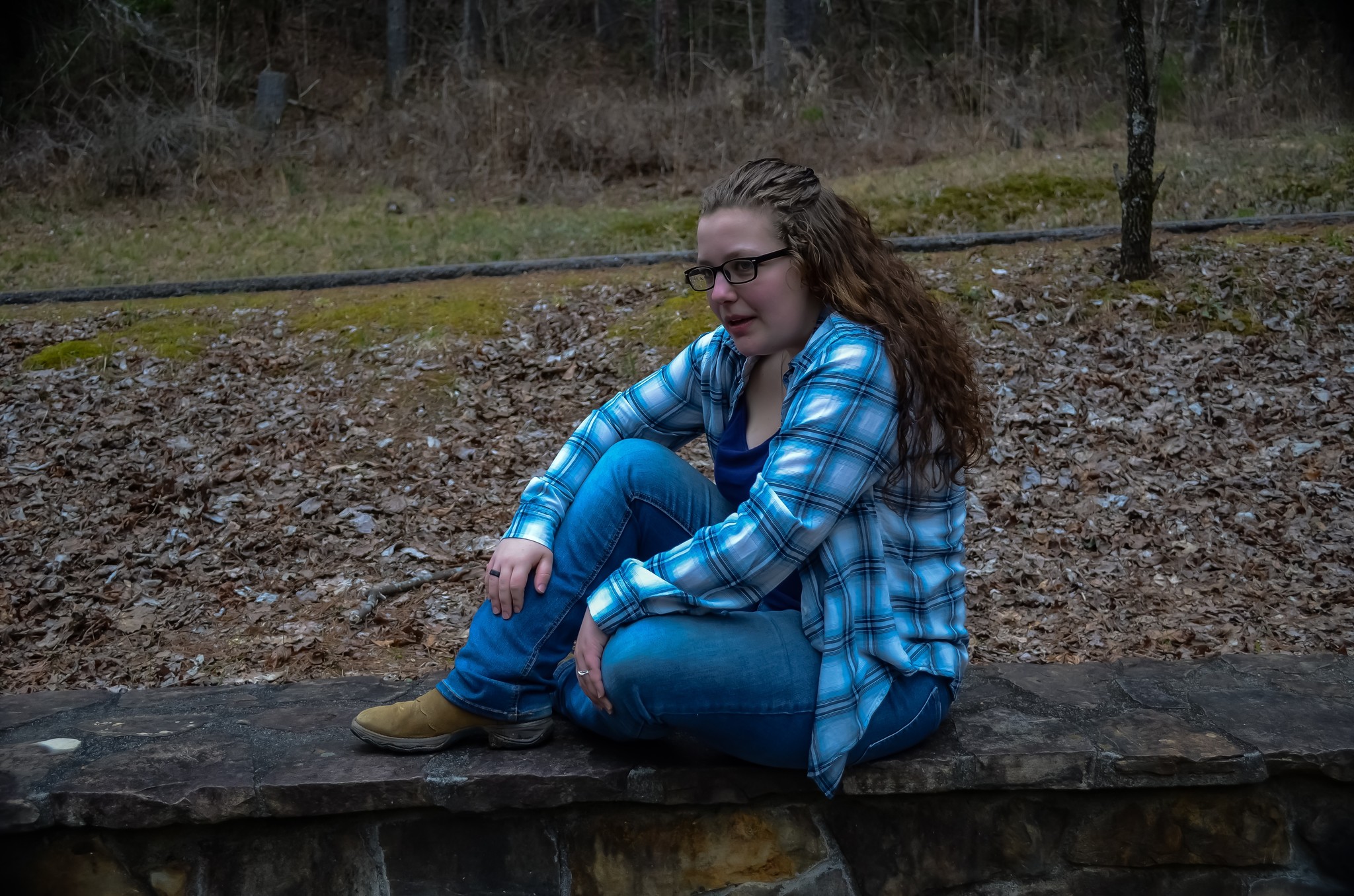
[489, 568, 501, 578]
[576, 670, 589, 678]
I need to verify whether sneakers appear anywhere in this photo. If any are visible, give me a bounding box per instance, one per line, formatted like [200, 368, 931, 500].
[349, 688, 554, 750]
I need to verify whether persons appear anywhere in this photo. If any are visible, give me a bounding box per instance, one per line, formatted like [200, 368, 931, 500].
[351, 158, 994, 794]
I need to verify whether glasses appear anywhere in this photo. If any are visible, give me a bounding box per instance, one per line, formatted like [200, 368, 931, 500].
[684, 245, 793, 292]
